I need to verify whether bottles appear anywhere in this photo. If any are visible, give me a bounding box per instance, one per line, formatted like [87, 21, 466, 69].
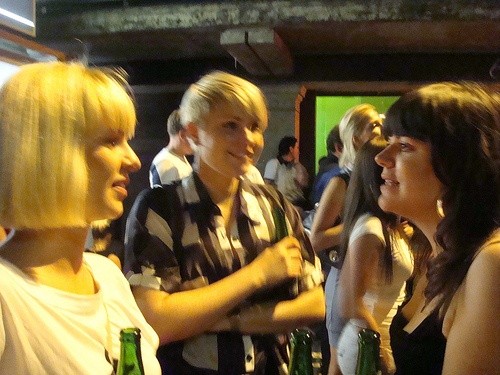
[287, 329, 314, 375]
[115, 328, 145, 375]
[354, 329, 383, 375]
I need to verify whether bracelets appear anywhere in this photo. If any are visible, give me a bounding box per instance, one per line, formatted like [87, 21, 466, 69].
[225, 304, 241, 336]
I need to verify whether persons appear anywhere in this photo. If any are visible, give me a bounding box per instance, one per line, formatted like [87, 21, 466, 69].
[149, 109, 196, 187]
[263, 135, 312, 208]
[374, 81, 500, 375]
[311, 103, 414, 375]
[304, 124, 345, 234]
[335, 140, 415, 375]
[123, 71, 328, 374]
[0, 62, 164, 375]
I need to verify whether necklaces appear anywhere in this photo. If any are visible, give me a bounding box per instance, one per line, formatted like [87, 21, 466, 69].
[418, 295, 432, 313]
